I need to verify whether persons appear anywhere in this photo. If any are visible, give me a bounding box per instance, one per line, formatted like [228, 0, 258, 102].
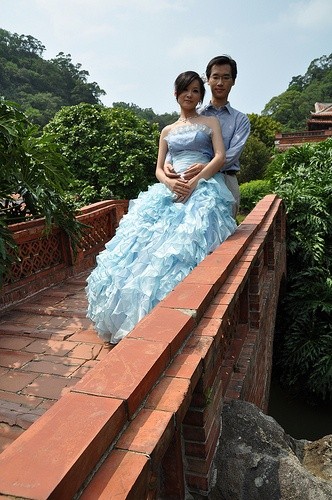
[84, 71, 235, 344]
[198, 53, 251, 228]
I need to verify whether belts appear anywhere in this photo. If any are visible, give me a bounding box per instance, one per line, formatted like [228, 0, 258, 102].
[222, 170, 239, 176]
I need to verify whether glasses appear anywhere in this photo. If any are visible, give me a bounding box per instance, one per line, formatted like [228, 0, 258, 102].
[210, 76, 232, 81]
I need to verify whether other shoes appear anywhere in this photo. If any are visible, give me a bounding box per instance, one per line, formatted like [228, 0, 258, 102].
[104, 341, 118, 349]
